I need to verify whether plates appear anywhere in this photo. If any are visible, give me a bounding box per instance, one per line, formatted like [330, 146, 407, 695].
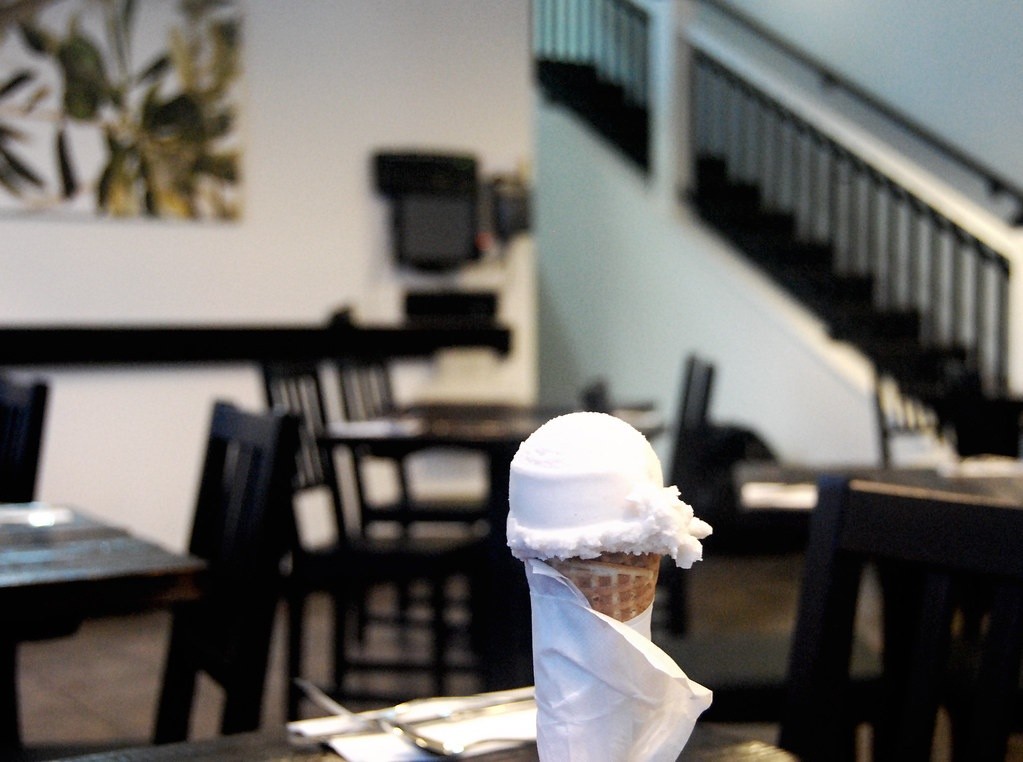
[394, 686, 538, 760]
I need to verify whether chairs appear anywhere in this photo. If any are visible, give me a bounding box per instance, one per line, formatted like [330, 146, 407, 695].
[2, 349, 1023, 762]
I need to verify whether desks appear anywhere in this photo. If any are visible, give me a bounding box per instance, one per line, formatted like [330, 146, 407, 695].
[368, 401, 667, 683]
[59, 690, 801, 762]
[735, 463, 1023, 742]
[0, 496, 210, 762]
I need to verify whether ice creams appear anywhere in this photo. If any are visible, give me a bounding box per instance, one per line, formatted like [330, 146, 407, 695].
[506, 412, 713, 622]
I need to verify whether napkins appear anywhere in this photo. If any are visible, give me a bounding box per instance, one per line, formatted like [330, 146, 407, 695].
[297, 700, 539, 761]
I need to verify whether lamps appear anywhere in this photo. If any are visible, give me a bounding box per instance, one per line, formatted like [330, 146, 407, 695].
[491, 169, 530, 242]
